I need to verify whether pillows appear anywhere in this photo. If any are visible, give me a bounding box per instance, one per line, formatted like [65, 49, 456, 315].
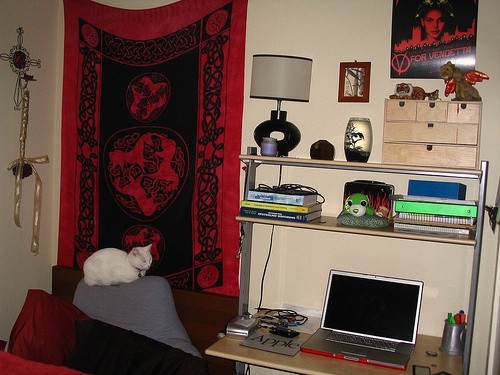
[4, 288, 209, 375]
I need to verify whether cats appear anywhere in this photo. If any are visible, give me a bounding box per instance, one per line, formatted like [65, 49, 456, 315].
[83, 244, 152, 285]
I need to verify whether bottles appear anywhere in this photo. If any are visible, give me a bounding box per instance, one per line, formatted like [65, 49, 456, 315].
[260, 137, 277, 157]
[310, 140, 335, 160]
[344, 117, 373, 163]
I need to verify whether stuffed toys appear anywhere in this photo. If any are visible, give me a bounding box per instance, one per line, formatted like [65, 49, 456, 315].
[336, 193, 374, 216]
[375, 205, 389, 217]
[389, 83, 438, 100]
[440, 61, 489, 101]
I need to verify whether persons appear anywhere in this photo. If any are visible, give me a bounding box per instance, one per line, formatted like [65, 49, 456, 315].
[417, 0, 453, 46]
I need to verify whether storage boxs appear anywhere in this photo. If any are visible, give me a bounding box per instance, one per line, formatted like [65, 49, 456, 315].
[409, 179, 467, 201]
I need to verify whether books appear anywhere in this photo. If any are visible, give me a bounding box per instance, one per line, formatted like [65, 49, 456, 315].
[239, 189, 323, 223]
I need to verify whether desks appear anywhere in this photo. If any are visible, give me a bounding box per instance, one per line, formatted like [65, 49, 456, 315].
[205, 317, 464, 375]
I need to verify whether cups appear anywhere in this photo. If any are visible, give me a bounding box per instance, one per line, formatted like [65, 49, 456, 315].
[439, 319, 466, 356]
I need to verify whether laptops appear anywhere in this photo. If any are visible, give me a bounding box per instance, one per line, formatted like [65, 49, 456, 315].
[300, 269, 424, 369]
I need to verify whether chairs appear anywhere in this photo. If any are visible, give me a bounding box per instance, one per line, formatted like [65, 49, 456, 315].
[71, 276, 201, 359]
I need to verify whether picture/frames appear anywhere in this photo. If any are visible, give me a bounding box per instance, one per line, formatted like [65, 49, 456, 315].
[338, 61, 371, 103]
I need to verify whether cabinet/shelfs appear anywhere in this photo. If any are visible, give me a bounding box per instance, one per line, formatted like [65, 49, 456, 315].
[237, 147, 489, 360]
[383, 98, 482, 169]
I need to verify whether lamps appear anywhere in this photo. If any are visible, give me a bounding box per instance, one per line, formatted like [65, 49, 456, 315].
[249, 54, 312, 156]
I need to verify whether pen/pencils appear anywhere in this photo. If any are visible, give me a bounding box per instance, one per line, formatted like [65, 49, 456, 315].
[448, 310, 468, 325]
